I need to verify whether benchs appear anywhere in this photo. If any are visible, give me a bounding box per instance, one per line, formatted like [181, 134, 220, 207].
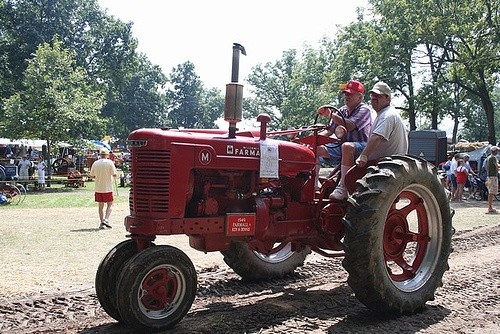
[64, 173, 92, 187]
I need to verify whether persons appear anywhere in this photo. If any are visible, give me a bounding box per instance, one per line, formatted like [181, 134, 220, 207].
[437, 146, 500, 214]
[90, 150, 117, 229]
[314, 80, 372, 200]
[0, 144, 125, 192]
[355, 82, 408, 168]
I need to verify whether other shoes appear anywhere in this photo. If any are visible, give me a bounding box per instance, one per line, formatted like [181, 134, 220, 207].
[489, 209, 496, 213]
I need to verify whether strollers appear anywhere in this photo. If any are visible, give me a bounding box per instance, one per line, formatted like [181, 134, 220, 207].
[472, 174, 500, 201]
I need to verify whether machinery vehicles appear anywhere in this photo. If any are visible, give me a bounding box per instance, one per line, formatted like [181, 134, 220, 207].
[95, 42, 455, 334]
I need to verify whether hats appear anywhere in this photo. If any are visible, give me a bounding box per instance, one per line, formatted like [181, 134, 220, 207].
[99, 148, 109, 155]
[491, 146, 500, 151]
[342, 81, 364, 94]
[368, 81, 391, 98]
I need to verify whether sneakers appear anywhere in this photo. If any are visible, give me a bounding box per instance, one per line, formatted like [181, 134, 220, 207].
[329, 184, 348, 201]
[102, 219, 112, 229]
[99, 224, 104, 229]
